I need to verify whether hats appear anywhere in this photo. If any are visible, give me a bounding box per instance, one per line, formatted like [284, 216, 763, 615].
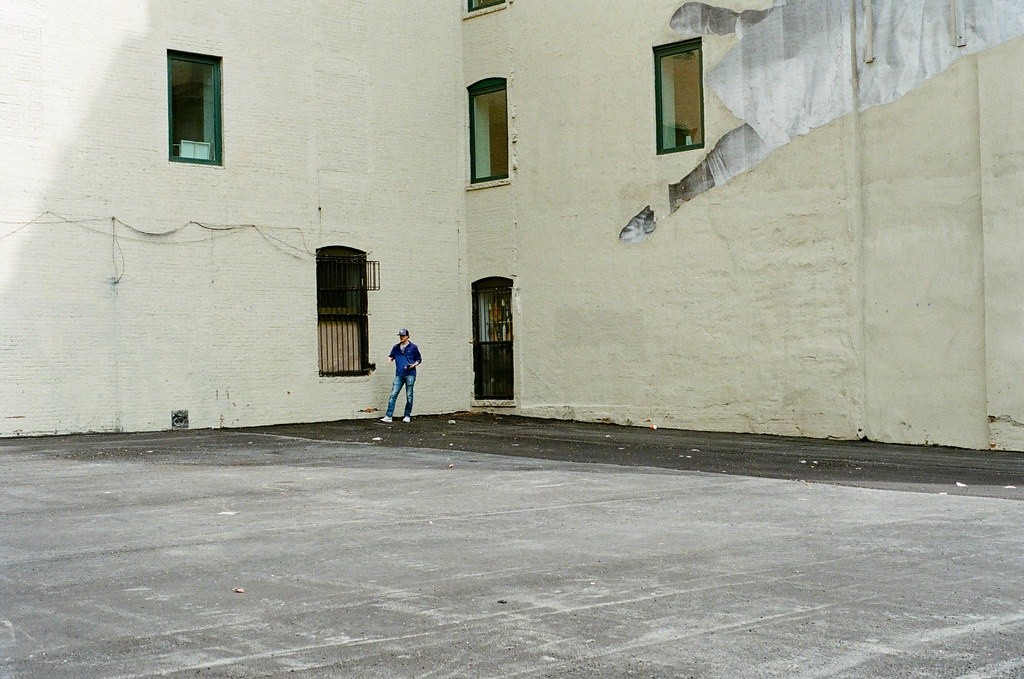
[397, 329, 409, 336]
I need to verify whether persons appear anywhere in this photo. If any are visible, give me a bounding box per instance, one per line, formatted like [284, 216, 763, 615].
[380, 329, 422, 422]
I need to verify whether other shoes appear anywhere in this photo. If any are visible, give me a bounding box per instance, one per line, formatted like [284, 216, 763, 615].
[402, 415, 410, 423]
[380, 416, 392, 422]
